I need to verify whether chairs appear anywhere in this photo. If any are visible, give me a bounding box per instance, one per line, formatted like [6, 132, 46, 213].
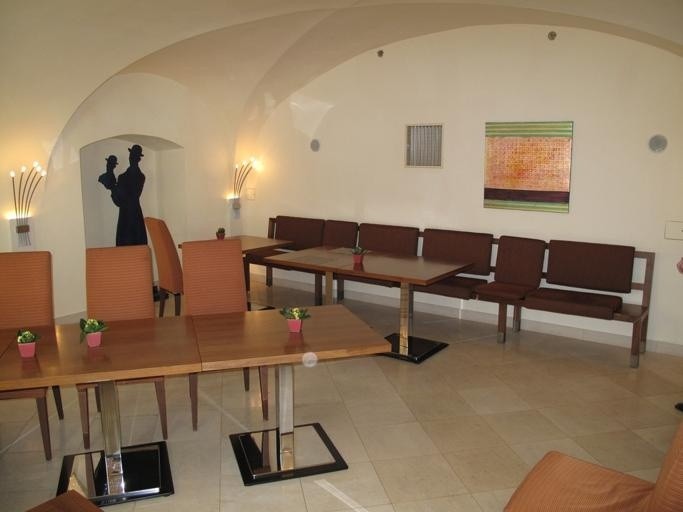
[0, 251, 63, 461]
[142, 216, 185, 317]
[242, 215, 655, 369]
[180, 239, 270, 431]
[75, 245, 171, 449]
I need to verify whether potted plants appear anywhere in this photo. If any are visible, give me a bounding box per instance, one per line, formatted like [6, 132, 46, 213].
[278, 304, 312, 333]
[215, 227, 225, 240]
[79, 316, 108, 347]
[15, 328, 38, 358]
[352, 246, 369, 264]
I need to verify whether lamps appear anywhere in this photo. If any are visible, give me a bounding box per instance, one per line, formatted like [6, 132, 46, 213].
[225, 158, 261, 219]
[4, 162, 46, 247]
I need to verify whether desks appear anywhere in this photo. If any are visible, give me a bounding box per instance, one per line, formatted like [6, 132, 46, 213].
[338, 246, 472, 364]
[0, 315, 191, 507]
[177, 235, 294, 311]
[191, 304, 392, 487]
[263, 244, 383, 305]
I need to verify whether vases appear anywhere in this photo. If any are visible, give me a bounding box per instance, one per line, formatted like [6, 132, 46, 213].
[353, 265, 363, 272]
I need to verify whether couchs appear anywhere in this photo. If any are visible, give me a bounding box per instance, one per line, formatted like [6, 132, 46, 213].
[499, 416, 683, 512]
